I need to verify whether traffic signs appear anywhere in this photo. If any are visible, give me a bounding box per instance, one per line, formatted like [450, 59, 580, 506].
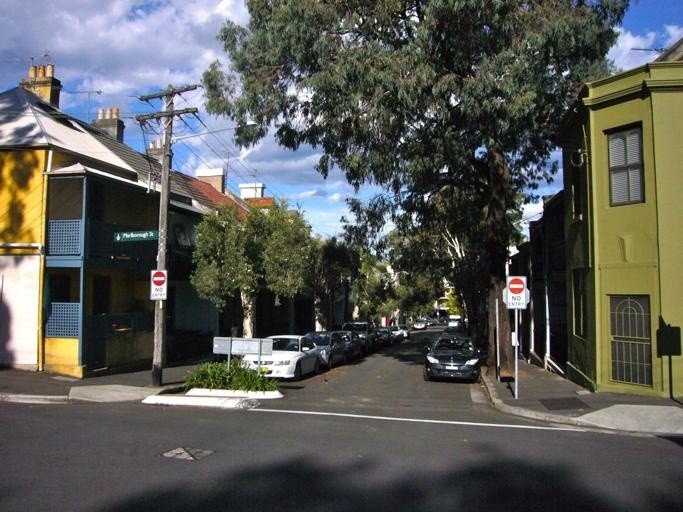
[115, 231, 157, 242]
[505, 277, 527, 294]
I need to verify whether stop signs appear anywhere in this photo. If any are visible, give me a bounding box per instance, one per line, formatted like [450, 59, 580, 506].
[150, 269, 167, 287]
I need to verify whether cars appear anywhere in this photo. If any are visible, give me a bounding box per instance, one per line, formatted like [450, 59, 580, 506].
[422, 333, 482, 384]
[308, 314, 449, 371]
[239, 335, 320, 382]
